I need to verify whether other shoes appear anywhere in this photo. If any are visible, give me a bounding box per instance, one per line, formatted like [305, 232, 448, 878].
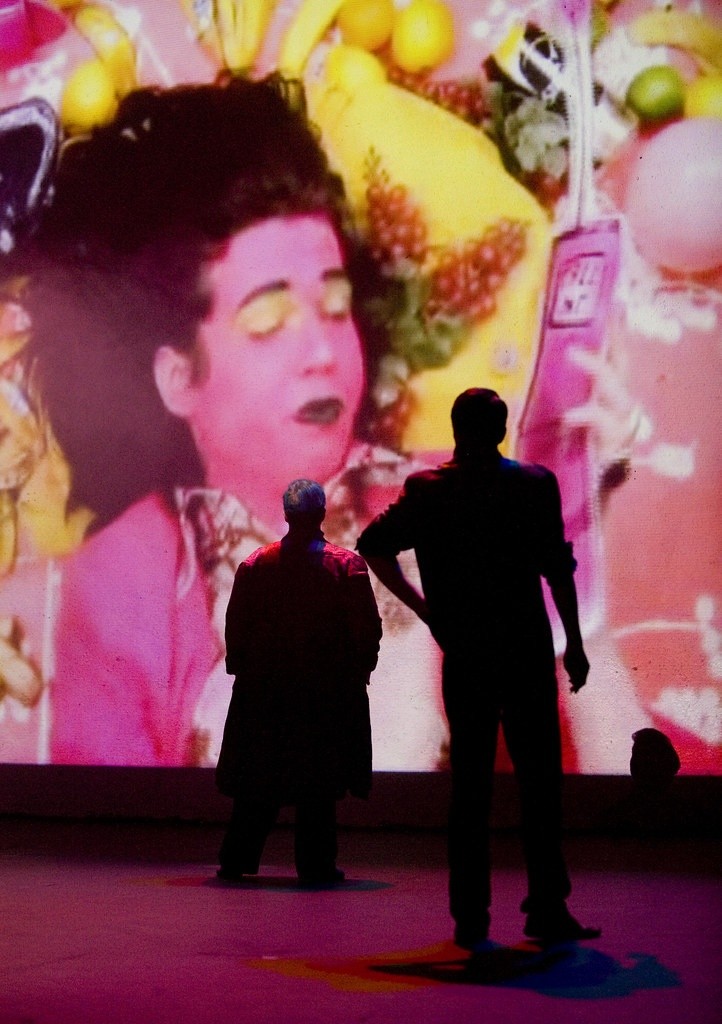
[216, 866, 244, 881]
[299, 868, 344, 890]
[455, 934, 504, 955]
[525, 898, 602, 940]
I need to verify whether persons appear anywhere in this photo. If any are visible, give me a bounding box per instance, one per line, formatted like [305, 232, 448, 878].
[17, 73, 655, 774]
[213, 479, 383, 887]
[355, 389, 602, 945]
[597, 729, 707, 837]
